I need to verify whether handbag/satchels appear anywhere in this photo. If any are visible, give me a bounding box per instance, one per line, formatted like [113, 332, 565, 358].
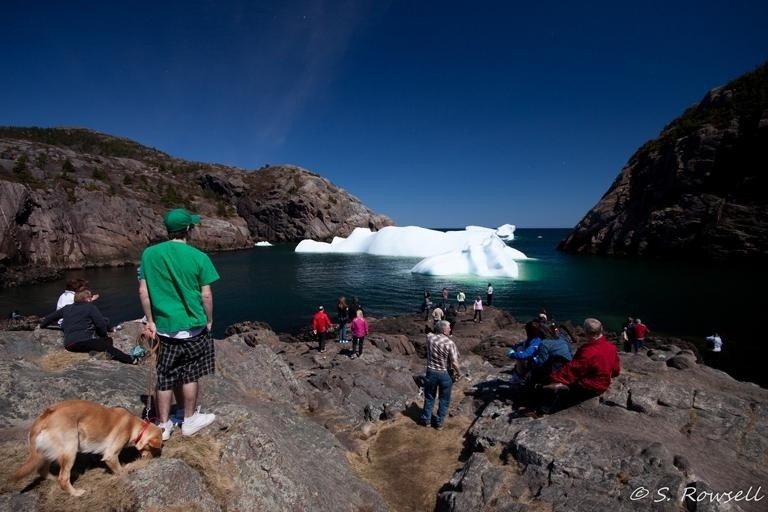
[706, 341, 714, 349]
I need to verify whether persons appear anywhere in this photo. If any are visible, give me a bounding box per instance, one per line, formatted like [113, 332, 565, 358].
[312, 305, 333, 354]
[349, 295, 366, 322]
[138, 208, 222, 441]
[705, 332, 723, 367]
[414, 280, 650, 431]
[39, 288, 146, 366]
[349, 309, 369, 360]
[337, 295, 350, 344]
[54, 275, 121, 332]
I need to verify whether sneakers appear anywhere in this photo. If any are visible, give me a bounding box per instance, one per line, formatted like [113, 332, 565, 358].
[158, 419, 174, 440]
[350, 354, 363, 359]
[182, 411, 216, 436]
[424, 422, 446, 430]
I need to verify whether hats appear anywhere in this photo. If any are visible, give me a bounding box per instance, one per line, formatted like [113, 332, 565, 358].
[164, 208, 202, 232]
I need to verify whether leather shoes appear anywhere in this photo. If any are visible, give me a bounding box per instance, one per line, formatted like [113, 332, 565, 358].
[542, 383, 569, 394]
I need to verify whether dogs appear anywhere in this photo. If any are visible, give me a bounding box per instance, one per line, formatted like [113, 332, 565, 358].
[12, 399, 165, 497]
